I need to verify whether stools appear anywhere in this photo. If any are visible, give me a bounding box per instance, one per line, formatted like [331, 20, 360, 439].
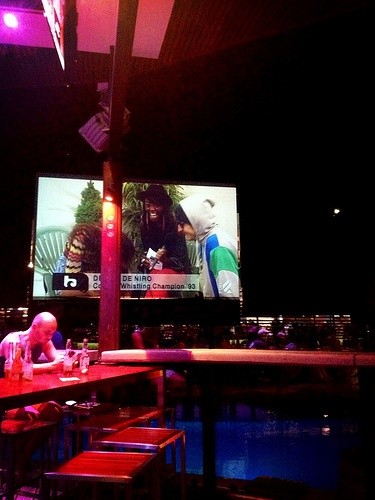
[0, 401, 187, 500]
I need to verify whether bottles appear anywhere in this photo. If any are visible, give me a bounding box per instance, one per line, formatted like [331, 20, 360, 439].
[63, 338, 73, 378]
[11, 341, 23, 380]
[22, 341, 33, 381]
[79, 339, 90, 376]
[3, 342, 14, 380]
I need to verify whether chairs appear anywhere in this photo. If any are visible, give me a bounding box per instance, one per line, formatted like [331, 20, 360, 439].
[33, 224, 70, 298]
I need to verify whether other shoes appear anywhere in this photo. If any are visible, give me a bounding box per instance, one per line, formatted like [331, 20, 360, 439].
[15, 469, 46, 486]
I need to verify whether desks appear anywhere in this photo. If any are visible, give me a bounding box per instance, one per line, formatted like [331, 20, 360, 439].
[0, 366, 167, 500]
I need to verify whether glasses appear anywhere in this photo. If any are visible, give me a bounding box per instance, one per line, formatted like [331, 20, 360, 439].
[176, 219, 190, 227]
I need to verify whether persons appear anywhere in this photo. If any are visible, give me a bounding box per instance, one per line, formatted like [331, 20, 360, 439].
[54, 185, 200, 297]
[0, 312, 65, 490]
[176, 194, 239, 297]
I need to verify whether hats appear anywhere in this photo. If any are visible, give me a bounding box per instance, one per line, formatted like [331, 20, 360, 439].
[136, 185, 173, 206]
[174, 205, 190, 224]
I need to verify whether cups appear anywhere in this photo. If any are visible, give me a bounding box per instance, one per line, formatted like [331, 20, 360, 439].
[119, 406, 131, 418]
